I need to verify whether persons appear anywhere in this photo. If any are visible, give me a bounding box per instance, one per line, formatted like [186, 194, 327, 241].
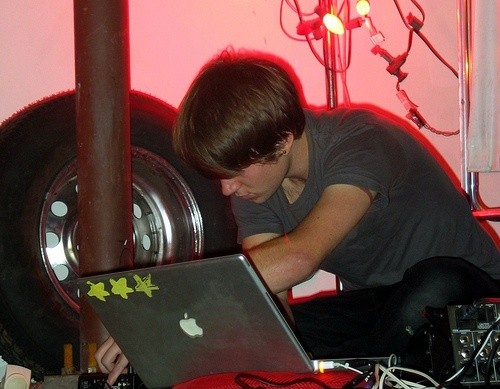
[95, 46, 500, 385]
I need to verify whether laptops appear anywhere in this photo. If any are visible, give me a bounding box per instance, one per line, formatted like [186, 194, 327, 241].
[77, 254, 401, 389]
